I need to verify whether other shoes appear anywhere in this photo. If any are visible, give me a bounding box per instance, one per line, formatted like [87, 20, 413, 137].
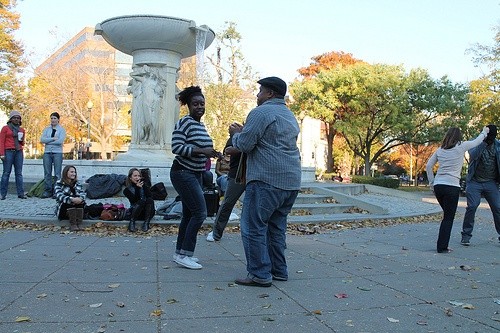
[437, 247, 454, 253]
[1, 191, 59, 200]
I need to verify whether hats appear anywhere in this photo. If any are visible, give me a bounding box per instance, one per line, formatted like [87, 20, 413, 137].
[9, 109, 22, 118]
[257, 75, 287, 96]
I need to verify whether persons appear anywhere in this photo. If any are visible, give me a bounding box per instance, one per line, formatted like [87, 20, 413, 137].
[78, 152, 94, 160]
[333, 163, 342, 177]
[205, 134, 248, 242]
[397, 172, 424, 188]
[0, 110, 27, 200]
[228, 76, 303, 289]
[53, 164, 87, 232]
[426, 124, 491, 253]
[202, 157, 213, 189]
[167, 85, 223, 271]
[215, 146, 232, 207]
[40, 112, 67, 199]
[460, 123, 500, 246]
[123, 168, 155, 232]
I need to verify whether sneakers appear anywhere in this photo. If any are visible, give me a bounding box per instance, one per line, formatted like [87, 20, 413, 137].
[207, 231, 215, 241]
[235, 276, 271, 287]
[271, 270, 289, 281]
[172, 252, 203, 270]
[460, 237, 471, 244]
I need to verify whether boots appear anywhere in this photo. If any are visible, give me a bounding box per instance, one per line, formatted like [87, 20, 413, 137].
[128, 204, 140, 231]
[67, 207, 85, 231]
[142, 203, 152, 231]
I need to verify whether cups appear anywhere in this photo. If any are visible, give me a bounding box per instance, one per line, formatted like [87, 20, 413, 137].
[18, 131, 23, 141]
